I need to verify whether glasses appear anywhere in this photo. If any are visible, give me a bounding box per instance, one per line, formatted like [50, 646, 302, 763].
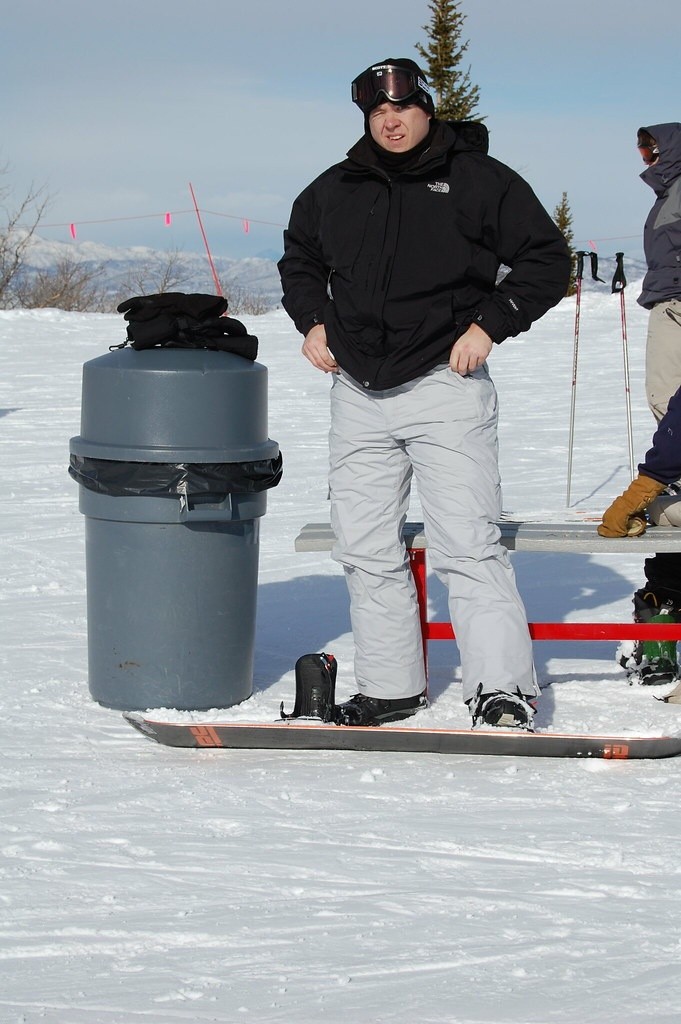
[639, 146, 661, 164]
[350, 65, 420, 107]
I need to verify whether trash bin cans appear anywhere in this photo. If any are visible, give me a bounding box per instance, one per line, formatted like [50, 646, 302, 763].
[67, 346, 280, 714]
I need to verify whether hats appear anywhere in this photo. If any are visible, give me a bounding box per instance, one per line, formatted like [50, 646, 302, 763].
[363, 58, 435, 121]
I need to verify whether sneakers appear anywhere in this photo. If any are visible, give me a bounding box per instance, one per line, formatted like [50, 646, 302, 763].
[468, 682, 537, 733]
[331, 691, 427, 726]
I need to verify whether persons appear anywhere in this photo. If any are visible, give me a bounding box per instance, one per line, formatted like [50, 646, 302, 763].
[638, 122, 681, 496]
[596, 385, 681, 685]
[276, 58, 573, 733]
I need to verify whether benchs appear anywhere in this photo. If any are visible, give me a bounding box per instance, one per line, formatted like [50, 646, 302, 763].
[296, 518, 681, 690]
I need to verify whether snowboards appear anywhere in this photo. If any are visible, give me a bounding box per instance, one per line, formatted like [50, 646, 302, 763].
[118, 709, 681, 761]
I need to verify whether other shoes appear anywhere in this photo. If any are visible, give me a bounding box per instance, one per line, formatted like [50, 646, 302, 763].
[617, 648, 676, 685]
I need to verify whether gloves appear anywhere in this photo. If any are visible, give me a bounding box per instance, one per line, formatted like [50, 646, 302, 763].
[598, 474, 668, 538]
[117, 291, 258, 362]
